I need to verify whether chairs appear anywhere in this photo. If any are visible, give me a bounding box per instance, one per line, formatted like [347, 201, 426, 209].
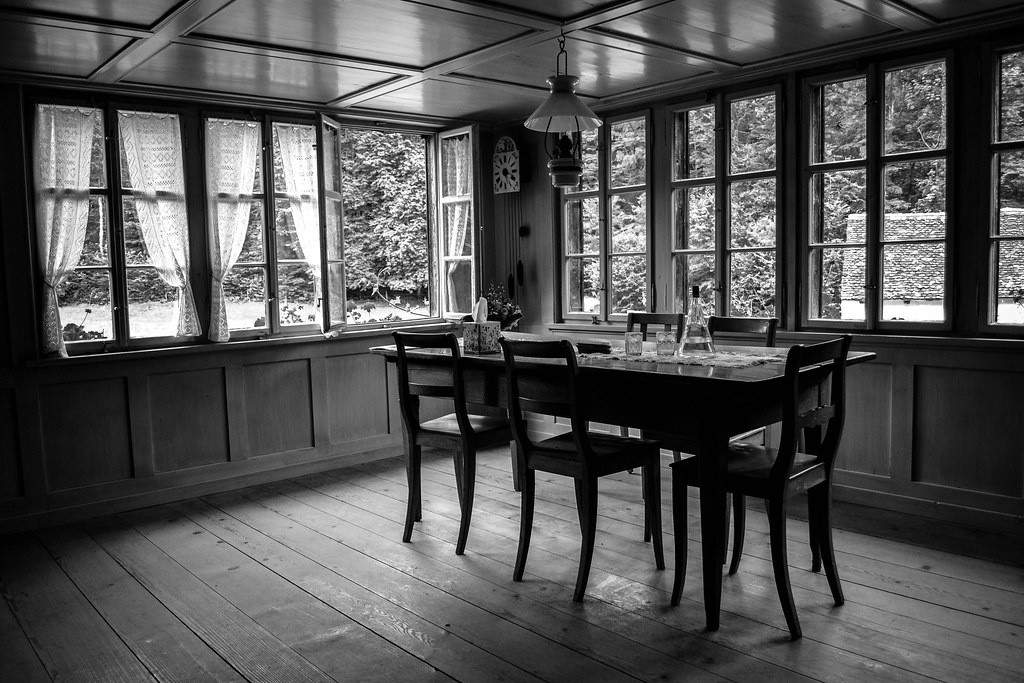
[393, 330, 528, 555]
[499, 339, 666, 602]
[671, 316, 778, 565]
[618, 313, 683, 474]
[668, 332, 852, 641]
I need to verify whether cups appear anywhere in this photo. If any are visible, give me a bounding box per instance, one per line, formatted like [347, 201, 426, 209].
[625, 332, 644, 356]
[655, 331, 677, 356]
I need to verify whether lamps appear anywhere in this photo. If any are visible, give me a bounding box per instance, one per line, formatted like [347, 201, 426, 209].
[524, 40, 603, 188]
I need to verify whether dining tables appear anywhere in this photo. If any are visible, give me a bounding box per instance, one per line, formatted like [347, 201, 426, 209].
[368, 332, 876, 630]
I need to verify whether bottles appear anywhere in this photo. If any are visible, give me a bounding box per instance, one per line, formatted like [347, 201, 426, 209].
[677, 285, 716, 359]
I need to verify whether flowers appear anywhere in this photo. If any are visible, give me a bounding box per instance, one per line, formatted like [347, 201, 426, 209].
[459, 282, 524, 331]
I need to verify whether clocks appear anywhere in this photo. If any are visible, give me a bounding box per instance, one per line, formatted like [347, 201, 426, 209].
[493, 136, 520, 195]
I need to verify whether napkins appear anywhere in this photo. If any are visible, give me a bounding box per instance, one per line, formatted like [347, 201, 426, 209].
[463, 297, 501, 355]
